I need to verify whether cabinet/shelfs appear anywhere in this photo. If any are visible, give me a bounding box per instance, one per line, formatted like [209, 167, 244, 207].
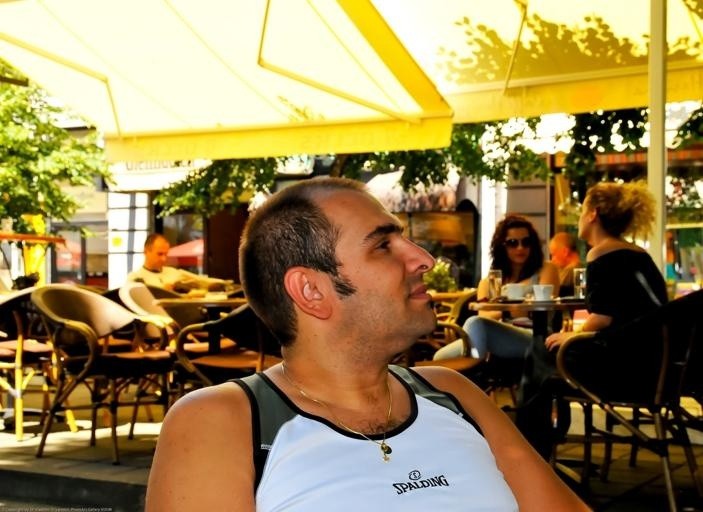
[506, 152, 703, 290]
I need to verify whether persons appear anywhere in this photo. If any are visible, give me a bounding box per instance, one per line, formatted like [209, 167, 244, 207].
[433, 215, 560, 360]
[515, 181, 667, 463]
[129, 233, 235, 292]
[548, 232, 580, 287]
[145, 179, 596, 511]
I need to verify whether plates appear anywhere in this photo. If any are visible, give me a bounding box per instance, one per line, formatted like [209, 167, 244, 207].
[497, 299, 525, 303]
[526, 299, 558, 303]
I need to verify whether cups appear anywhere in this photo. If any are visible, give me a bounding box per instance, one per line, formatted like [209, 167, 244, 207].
[533, 284, 554, 300]
[504, 283, 526, 300]
[573, 268, 587, 299]
[488, 270, 502, 302]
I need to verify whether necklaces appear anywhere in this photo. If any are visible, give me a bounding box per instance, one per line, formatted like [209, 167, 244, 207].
[280, 359, 393, 465]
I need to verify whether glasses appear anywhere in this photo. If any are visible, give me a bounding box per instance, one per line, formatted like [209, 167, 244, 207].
[503, 237, 531, 247]
